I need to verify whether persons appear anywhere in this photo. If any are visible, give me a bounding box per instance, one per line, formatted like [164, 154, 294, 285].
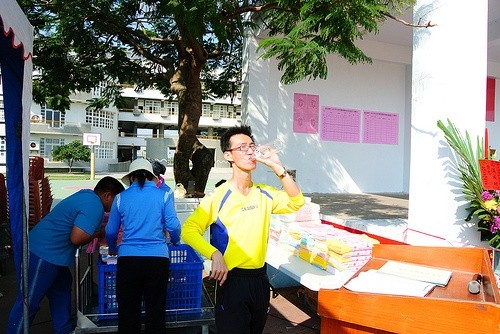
[8, 176, 126, 334]
[105, 156, 182, 334]
[180, 124, 305, 334]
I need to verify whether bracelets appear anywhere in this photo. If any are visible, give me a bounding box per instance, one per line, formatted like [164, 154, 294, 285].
[276, 166, 287, 178]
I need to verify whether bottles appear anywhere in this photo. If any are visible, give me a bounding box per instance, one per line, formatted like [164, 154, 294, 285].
[251, 140, 286, 159]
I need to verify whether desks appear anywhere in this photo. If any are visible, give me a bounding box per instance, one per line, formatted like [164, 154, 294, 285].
[265, 213, 412, 293]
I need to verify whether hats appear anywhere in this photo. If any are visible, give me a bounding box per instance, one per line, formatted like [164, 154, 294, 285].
[121, 158, 159, 185]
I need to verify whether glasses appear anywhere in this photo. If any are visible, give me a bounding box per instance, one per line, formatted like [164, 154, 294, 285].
[226, 144, 256, 151]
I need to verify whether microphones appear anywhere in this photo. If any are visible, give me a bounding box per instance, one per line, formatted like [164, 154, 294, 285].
[468, 274, 482, 293]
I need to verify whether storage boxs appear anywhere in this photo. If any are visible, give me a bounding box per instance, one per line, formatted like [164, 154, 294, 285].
[97, 244, 204, 319]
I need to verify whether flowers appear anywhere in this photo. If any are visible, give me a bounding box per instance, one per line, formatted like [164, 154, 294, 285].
[480, 187, 500, 235]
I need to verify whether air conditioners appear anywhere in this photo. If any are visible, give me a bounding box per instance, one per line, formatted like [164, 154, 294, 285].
[30, 140, 39, 151]
[30, 113, 43, 123]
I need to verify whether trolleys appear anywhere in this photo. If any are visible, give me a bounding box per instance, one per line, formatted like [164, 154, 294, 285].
[73, 242, 217, 334]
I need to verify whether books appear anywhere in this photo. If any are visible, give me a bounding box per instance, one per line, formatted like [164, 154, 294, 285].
[342, 261, 453, 297]
